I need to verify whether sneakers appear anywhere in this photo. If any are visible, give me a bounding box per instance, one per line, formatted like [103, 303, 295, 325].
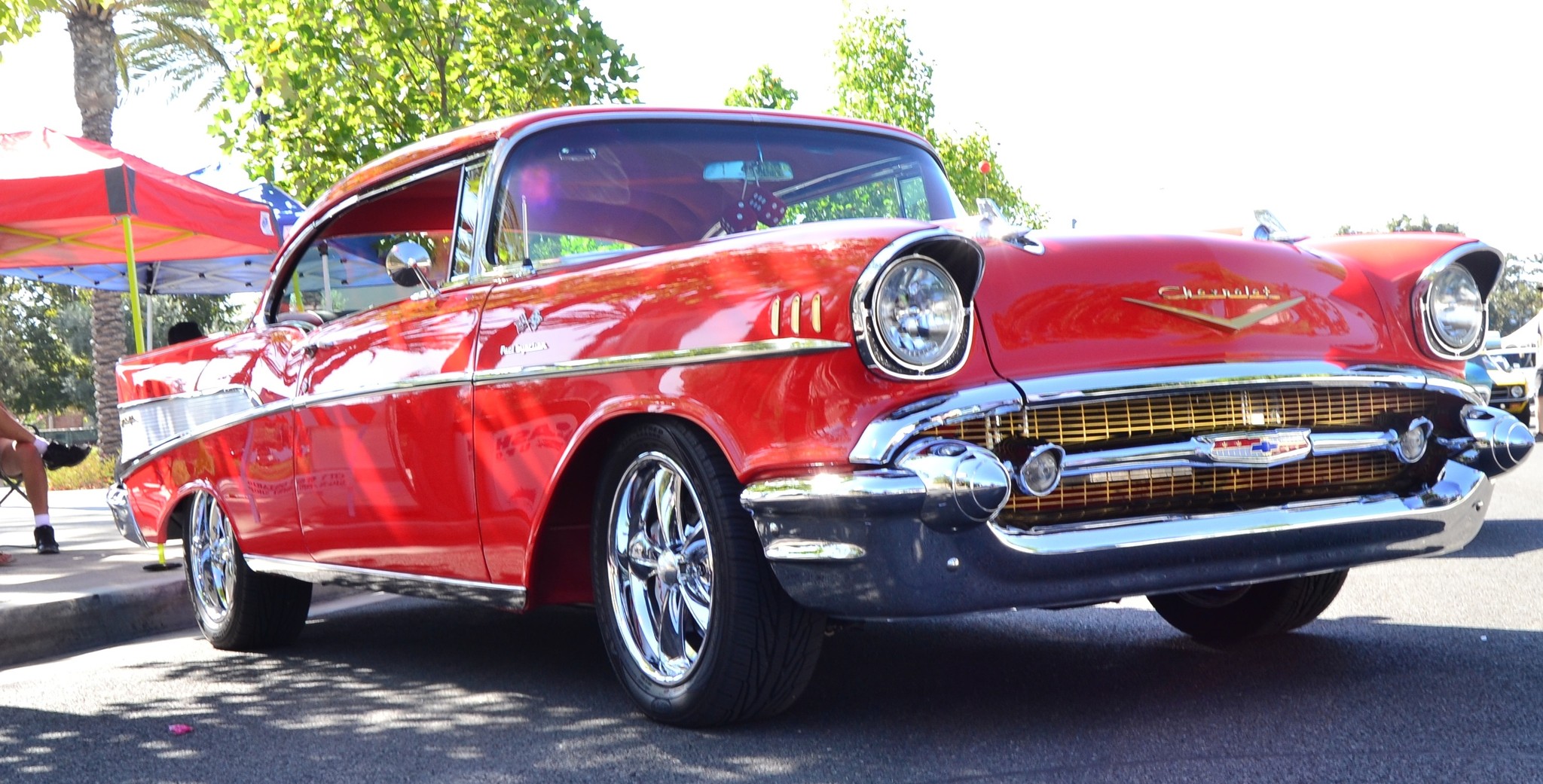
[33, 524, 60, 554]
[41, 439, 91, 472]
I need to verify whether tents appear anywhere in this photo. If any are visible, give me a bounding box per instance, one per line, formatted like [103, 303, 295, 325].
[2, 155, 398, 354]
[2, 128, 281, 571]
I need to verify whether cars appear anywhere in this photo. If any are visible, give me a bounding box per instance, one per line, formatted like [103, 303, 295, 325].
[1463, 327, 1543, 442]
[106, 105, 1535, 733]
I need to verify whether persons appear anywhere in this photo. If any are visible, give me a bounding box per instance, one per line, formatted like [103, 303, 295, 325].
[0, 400, 93, 554]
